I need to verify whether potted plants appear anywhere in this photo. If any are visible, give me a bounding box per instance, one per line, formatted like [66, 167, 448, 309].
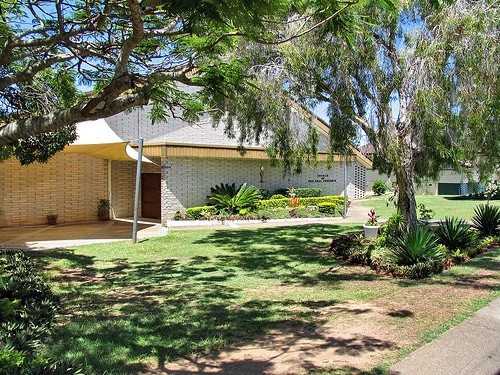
[363, 208, 381, 241]
[97, 198, 113, 221]
[174, 210, 182, 221]
[46, 211, 58, 226]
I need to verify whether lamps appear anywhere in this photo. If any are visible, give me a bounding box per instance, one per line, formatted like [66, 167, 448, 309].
[259, 165, 265, 184]
